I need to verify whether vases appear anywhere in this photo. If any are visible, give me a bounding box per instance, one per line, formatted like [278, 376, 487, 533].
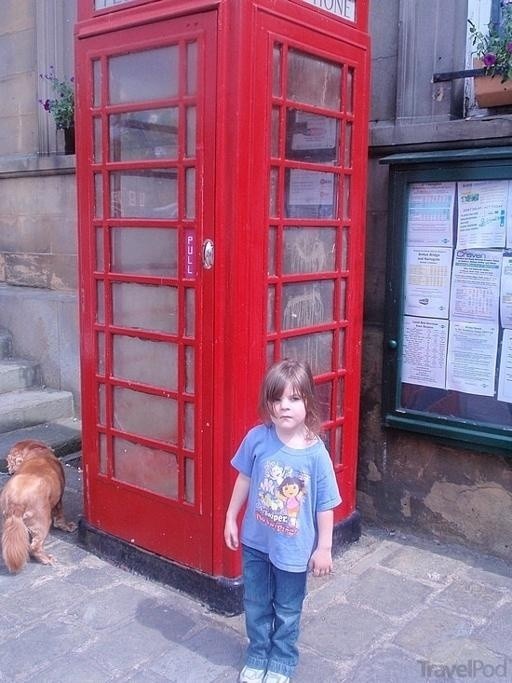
[468, 52, 512, 108]
[64, 127, 75, 153]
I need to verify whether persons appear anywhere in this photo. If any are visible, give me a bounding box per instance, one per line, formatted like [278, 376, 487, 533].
[224, 357, 343, 683]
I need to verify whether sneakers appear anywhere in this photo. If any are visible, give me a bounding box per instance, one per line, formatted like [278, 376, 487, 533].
[239, 665, 289, 683]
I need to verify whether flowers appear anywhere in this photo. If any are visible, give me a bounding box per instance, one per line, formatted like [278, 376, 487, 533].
[460, 0, 512, 80]
[39, 65, 73, 130]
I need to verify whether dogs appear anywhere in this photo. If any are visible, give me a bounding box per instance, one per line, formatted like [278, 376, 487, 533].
[0, 438, 79, 575]
